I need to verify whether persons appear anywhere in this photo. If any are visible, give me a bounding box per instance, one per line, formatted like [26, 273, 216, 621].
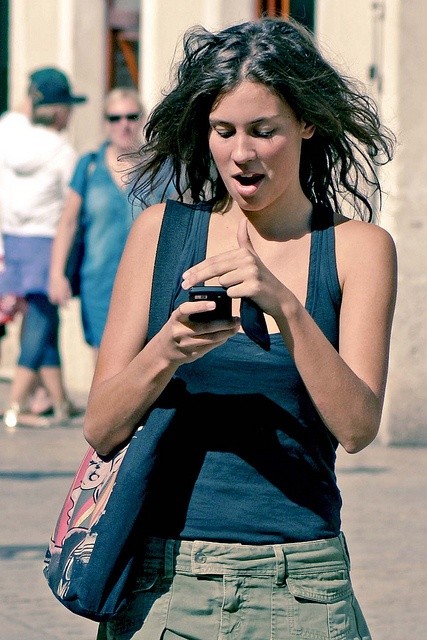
[47, 86, 182, 394]
[83, 18, 397, 640]
[0, 65, 86, 429]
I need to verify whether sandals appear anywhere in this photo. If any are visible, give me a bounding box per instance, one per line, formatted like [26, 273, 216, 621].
[4, 406, 59, 429]
[54, 397, 85, 421]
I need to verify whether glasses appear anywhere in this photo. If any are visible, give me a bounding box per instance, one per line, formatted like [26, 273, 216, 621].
[103, 110, 140, 124]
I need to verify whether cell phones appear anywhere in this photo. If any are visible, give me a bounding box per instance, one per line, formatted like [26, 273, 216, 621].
[188, 285, 233, 324]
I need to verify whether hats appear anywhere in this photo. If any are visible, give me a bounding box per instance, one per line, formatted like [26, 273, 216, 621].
[27, 67, 86, 106]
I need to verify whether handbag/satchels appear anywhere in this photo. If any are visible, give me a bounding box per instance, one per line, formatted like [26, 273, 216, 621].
[64, 220, 85, 296]
[42, 375, 187, 624]
